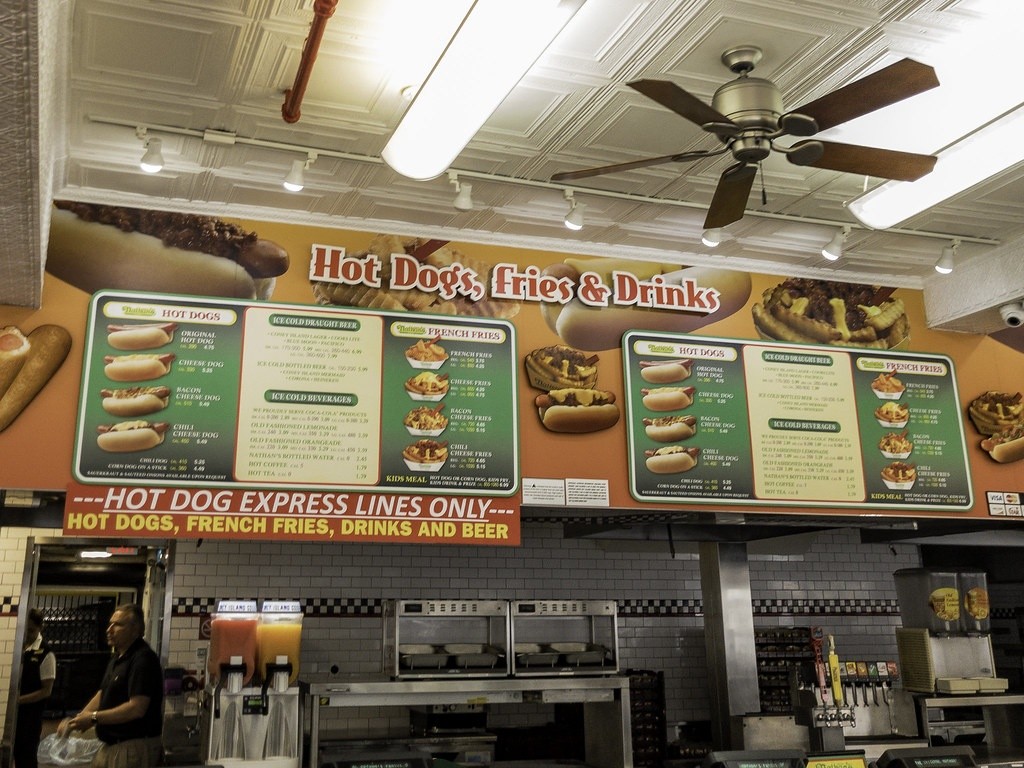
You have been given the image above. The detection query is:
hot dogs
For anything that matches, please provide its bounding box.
[539,256,753,351]
[981,425,1024,464]
[638,358,700,473]
[534,388,620,433]
[45,201,290,302]
[96,322,178,453]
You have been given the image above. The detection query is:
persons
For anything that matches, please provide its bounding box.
[11,610,56,768]
[59,604,167,768]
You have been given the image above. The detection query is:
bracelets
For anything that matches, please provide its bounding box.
[91,709,99,725]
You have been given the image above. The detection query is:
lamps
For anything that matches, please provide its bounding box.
[448,173,475,214]
[135,127,166,175]
[282,152,319,194]
[559,193,588,233]
[820,226,854,262]
[934,240,965,276]
[700,224,725,250]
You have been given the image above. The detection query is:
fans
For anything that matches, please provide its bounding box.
[547,41,944,234]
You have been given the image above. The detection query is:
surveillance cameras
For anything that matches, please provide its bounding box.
[1000,303,1024,328]
